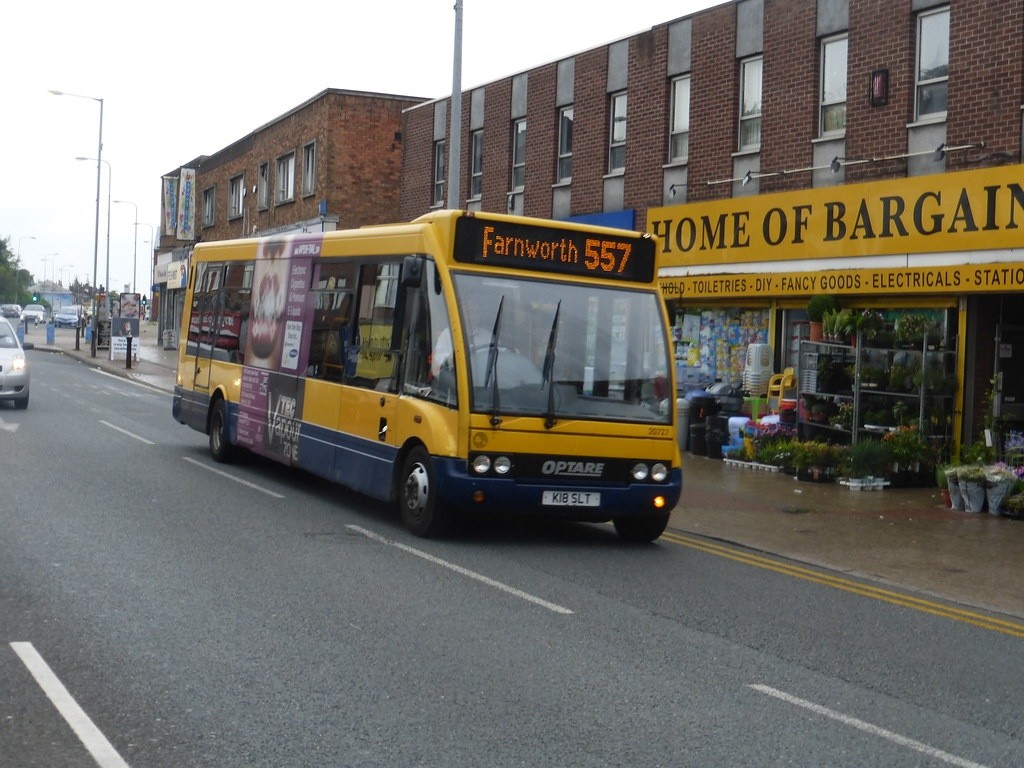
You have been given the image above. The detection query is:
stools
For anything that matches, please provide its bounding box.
[743,359,769,392]
[743,353,769,386]
[743,348,773,378]
[744,363,768,395]
[743,344,774,375]
[743,350,772,383]
[743,356,769,389]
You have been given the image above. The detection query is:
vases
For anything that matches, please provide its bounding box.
[898,340,940,351]
[886,458,913,483]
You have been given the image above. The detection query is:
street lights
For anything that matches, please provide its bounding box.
[49,89,104,351]
[13,236,36,303]
[134,222,154,321]
[76,156,112,321]
[113,199,138,343]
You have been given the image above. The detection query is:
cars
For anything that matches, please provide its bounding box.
[0,314,34,410]
[0,303,98,329]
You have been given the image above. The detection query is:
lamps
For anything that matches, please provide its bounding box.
[742,170,784,187]
[932,143,981,161]
[669,182,707,199]
[831,156,873,173]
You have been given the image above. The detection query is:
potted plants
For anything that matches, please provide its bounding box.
[723,293,1024,519]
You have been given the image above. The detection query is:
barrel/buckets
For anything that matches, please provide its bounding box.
[779,398,797,423]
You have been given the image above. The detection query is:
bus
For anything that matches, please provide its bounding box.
[172,206,684,546]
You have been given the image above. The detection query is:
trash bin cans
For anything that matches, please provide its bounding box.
[685,390,714,450]
[704,416,729,459]
[660,398,690,452]
[705,382,731,398]
[689,423,707,455]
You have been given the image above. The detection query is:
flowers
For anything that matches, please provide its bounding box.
[743,436,762,460]
[944,466,966,510]
[900,312,943,343]
[983,465,1018,516]
[830,401,853,424]
[931,416,951,467]
[956,466,988,513]
[880,424,943,466]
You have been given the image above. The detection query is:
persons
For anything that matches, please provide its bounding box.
[181,265,186,287]
[430,303,520,377]
[119,321,132,337]
[82,313,88,327]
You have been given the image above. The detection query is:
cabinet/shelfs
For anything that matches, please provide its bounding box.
[854,332,958,444]
[796,317,898,445]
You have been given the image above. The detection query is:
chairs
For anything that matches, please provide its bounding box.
[769,367,798,404]
[767,366,799,406]
